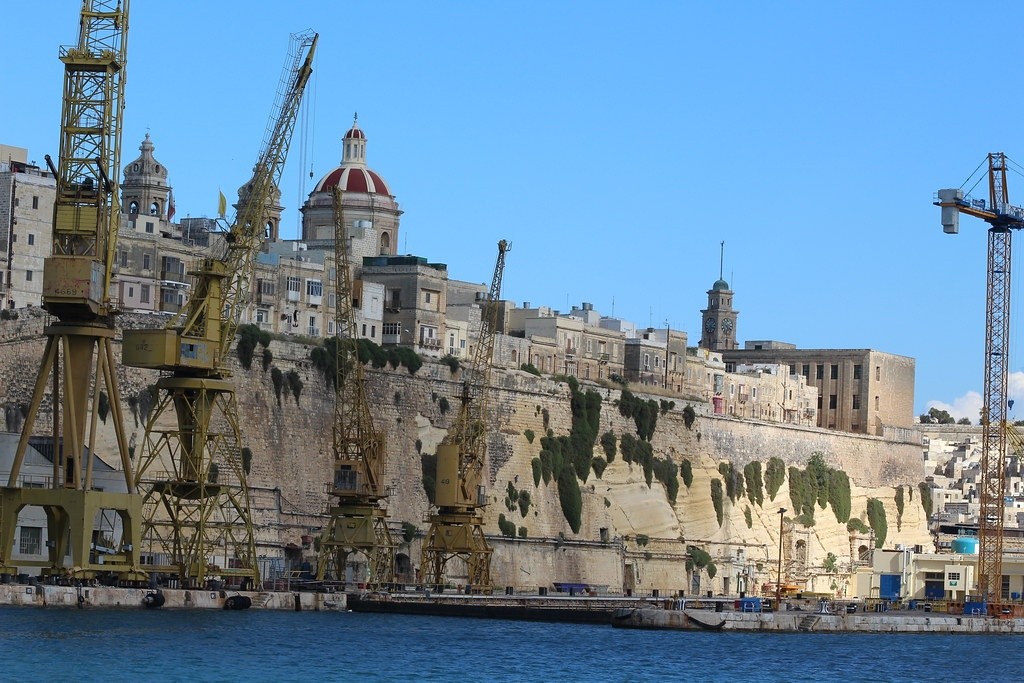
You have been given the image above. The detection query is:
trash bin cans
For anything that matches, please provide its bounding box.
[739,598,760,613]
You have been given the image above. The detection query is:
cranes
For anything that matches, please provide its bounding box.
[931,151,1024,617]
